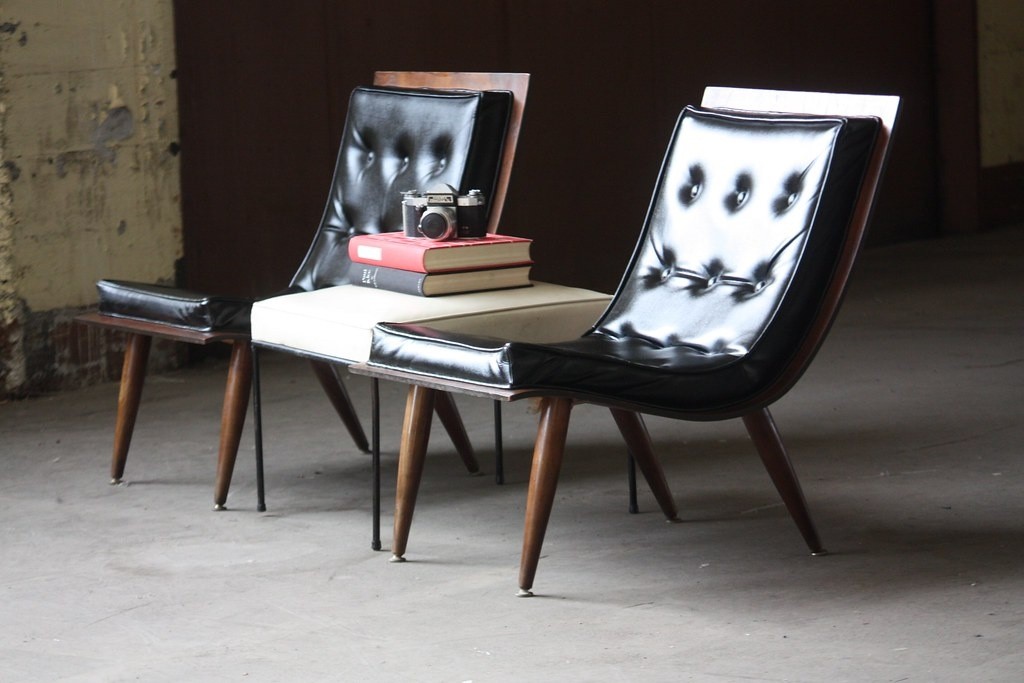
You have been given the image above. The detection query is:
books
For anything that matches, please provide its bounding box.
[348,262,533,296]
[348,231,535,273]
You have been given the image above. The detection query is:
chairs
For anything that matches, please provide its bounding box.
[73,71,535,514]
[349,86,902,598]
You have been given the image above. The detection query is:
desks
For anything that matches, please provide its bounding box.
[249,279,638,551]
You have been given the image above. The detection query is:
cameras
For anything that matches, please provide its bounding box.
[401,182,487,242]
